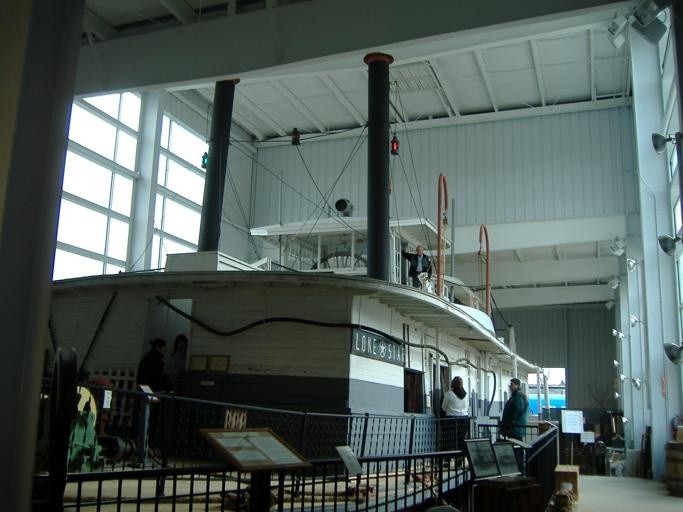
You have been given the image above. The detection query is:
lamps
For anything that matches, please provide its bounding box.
[390,81,399,156]
[656,231,681,255]
[605,239,645,426]
[200,152,208,168]
[651,133,678,152]
[661,340,682,364]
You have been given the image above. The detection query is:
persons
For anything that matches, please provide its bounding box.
[441,375,470,467]
[401,244,432,287]
[136,334,189,392]
[500,378,527,476]
